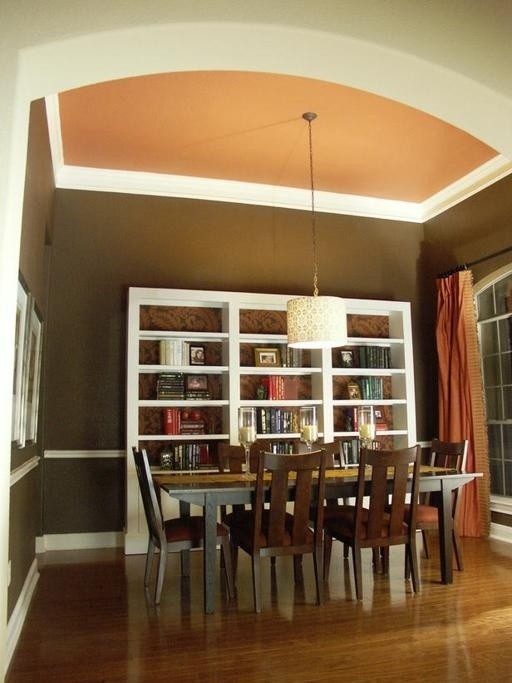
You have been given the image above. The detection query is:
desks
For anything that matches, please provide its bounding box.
[148,462,486,615]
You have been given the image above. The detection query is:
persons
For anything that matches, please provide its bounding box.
[261,355,273,362]
[343,353,352,367]
[186,376,201,388]
[194,349,203,361]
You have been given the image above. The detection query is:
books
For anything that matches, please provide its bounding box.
[171,443,219,470]
[258,374,298,454]
[184,391,214,401]
[358,345,392,369]
[157,338,190,366]
[160,407,205,435]
[343,405,386,466]
[358,375,384,400]
[278,343,304,367]
[154,373,185,400]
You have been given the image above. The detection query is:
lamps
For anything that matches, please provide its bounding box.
[286,111,348,351]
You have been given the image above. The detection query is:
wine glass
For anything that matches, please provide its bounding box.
[298,405,317,454]
[236,405,258,478]
[358,404,376,450]
